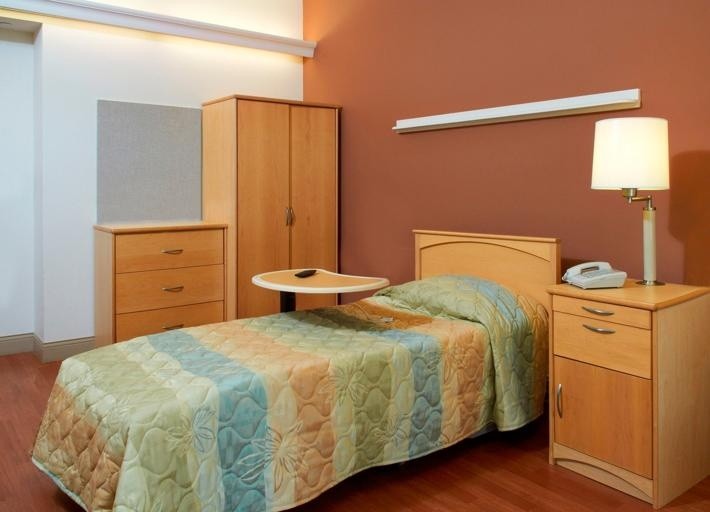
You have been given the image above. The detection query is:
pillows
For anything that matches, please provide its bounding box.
[377,276,544,322]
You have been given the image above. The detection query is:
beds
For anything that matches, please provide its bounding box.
[31,228,561,511]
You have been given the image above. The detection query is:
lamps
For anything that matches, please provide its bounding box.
[591,116,671,286]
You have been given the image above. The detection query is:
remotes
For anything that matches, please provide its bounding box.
[294,270,318,277]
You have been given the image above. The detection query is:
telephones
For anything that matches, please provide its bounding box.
[561,261,627,289]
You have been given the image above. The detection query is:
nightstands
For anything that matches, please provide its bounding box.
[549,276,710,509]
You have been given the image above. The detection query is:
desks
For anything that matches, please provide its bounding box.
[252,269,390,313]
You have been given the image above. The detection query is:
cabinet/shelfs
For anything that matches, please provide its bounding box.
[92,222,228,348]
[201,95,341,321]
